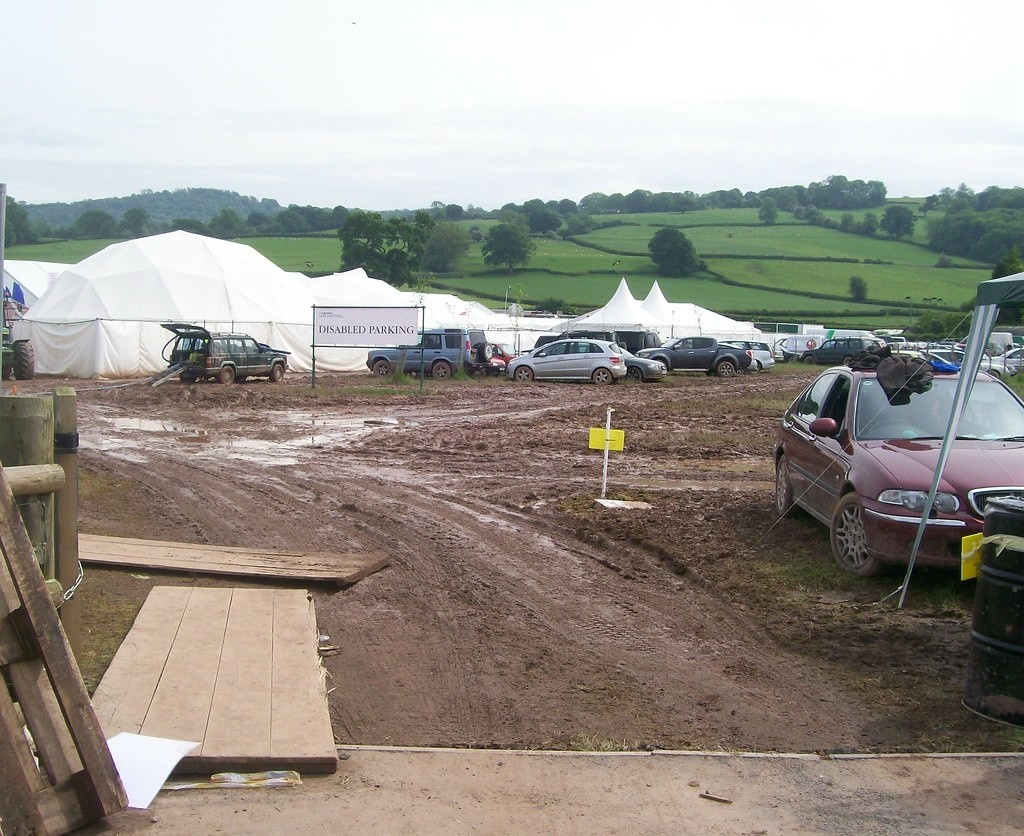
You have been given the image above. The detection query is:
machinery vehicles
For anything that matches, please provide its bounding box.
[2,291,35,380]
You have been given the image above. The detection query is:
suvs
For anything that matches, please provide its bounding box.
[800,338,885,365]
[366,329,493,379]
[160,324,290,386]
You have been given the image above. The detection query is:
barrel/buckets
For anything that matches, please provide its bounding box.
[960,495,1024,729]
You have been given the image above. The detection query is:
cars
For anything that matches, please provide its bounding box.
[772,365,1024,578]
[488,343,517,373]
[879,335,1024,380]
[771,344,784,361]
[506,339,627,385]
[718,341,775,374]
[619,347,668,382]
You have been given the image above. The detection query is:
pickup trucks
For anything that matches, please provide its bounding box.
[634,337,752,378]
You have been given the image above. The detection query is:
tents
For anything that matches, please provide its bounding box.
[2,229,762,378]
